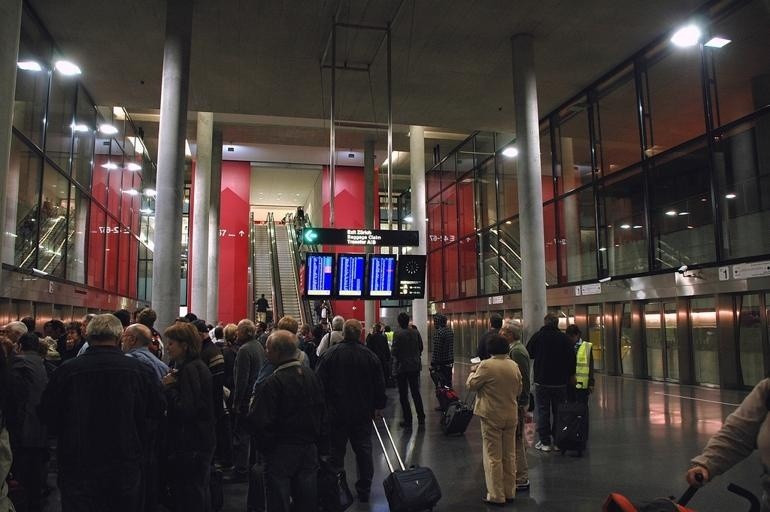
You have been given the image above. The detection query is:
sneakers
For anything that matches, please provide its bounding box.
[535,441,551,452]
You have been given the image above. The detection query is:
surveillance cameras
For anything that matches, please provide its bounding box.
[678,265,688,274]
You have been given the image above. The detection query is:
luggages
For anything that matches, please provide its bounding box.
[440,392,478,437]
[554,382,586,457]
[371,414,442,512]
[429,367,458,411]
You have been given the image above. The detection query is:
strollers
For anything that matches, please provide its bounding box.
[603,473,760,512]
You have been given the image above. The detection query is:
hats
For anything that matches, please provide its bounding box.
[190,320,209,332]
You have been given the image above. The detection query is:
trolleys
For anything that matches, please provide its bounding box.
[429,368,462,409]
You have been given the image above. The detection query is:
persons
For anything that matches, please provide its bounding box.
[429,311,455,412]
[476,313,502,361]
[296,205,316,262]
[526,314,575,451]
[314,299,326,317]
[18,196,56,243]
[497,319,530,491]
[465,335,523,507]
[253,293,270,322]
[564,324,594,436]
[317,302,331,316]
[0,310,424,512]
[687,377,769,511]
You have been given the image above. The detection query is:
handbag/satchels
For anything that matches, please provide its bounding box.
[317,466,353,511]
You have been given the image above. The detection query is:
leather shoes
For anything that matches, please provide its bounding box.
[222,471,250,483]
